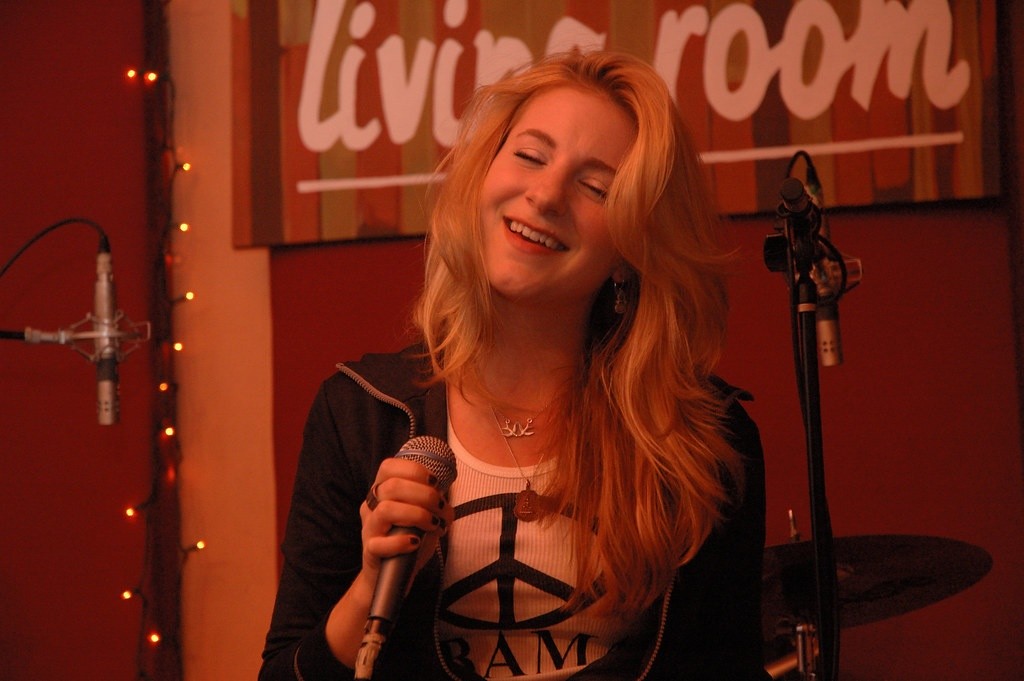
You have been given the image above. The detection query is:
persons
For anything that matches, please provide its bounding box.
[258,53,765,680]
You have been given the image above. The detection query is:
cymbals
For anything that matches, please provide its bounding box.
[761,533,993,635]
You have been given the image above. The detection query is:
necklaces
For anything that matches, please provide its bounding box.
[485,398,556,523]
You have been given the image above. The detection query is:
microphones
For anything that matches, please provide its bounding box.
[354,437,458,681]
[93,235,119,425]
[804,167,843,366]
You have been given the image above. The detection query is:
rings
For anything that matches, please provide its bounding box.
[366,483,378,510]
